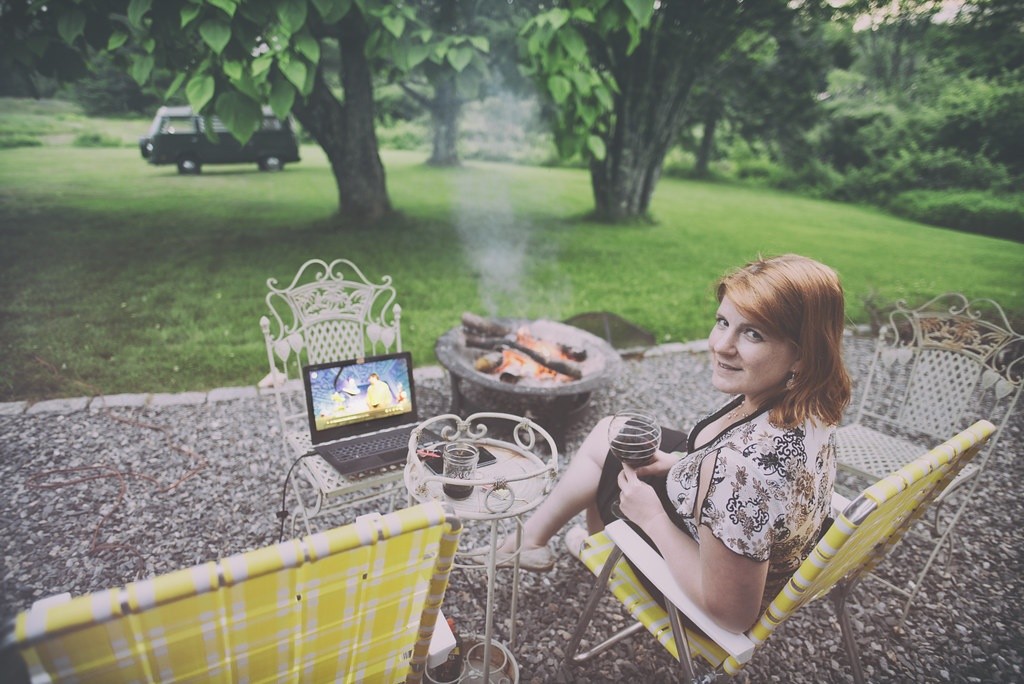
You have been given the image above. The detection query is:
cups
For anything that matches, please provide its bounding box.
[442,443,479,501]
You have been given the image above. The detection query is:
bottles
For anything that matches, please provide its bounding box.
[435,617,463,684]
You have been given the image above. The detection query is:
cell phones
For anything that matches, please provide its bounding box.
[425,446,496,476]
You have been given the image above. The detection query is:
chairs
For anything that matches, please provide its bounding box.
[564,420,997,684]
[829,293,1024,640]
[0,497,457,684]
[260,258,415,538]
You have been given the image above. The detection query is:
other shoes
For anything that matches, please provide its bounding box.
[564,525,590,558]
[468,536,556,573]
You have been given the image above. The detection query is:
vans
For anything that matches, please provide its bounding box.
[140,103,302,175]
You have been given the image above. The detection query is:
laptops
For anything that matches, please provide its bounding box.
[303,351,448,477]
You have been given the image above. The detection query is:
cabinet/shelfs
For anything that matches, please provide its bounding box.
[402,411,558,684]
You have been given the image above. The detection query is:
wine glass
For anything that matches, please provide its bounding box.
[608,409,662,522]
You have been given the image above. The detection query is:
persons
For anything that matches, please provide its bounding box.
[365,374,392,409]
[466,250,852,636]
